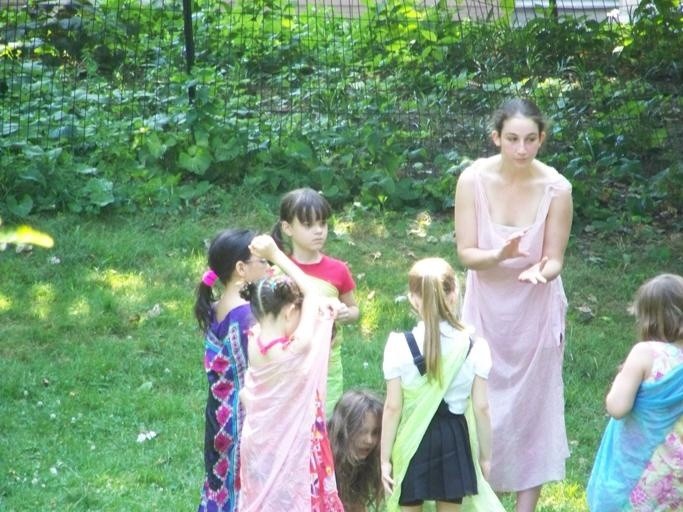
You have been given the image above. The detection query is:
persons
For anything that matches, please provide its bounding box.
[266,185,360,419]
[379,258,505,512]
[194,229,270,512]
[323,386,386,512]
[583,274,683,512]
[235,235,346,512]
[454,96,574,512]
